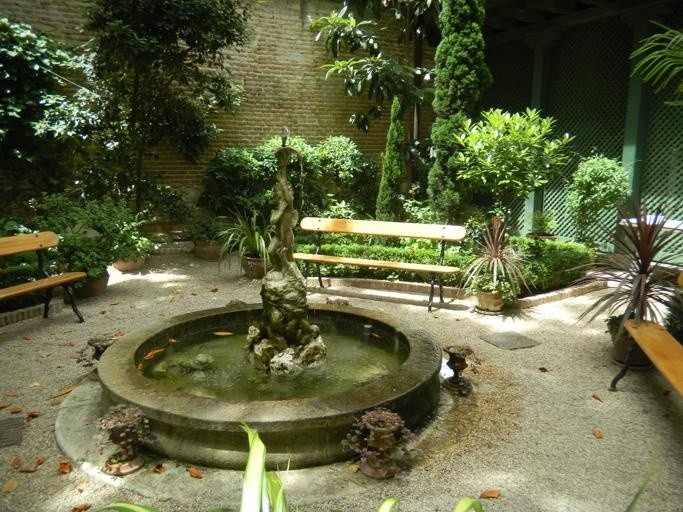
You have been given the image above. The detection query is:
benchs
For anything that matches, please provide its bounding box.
[292,215,467,312]
[1,230,87,323]
[609,273,682,396]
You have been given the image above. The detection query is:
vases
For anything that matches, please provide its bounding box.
[111,433,139,459]
[350,441,398,477]
[444,347,474,391]
[87,338,113,360]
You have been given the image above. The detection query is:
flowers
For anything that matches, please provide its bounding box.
[344,406,421,476]
[93,404,155,460]
[442,342,482,396]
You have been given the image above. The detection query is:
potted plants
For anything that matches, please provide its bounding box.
[455,216,538,315]
[189,205,277,279]
[68,217,151,298]
[565,196,683,371]
